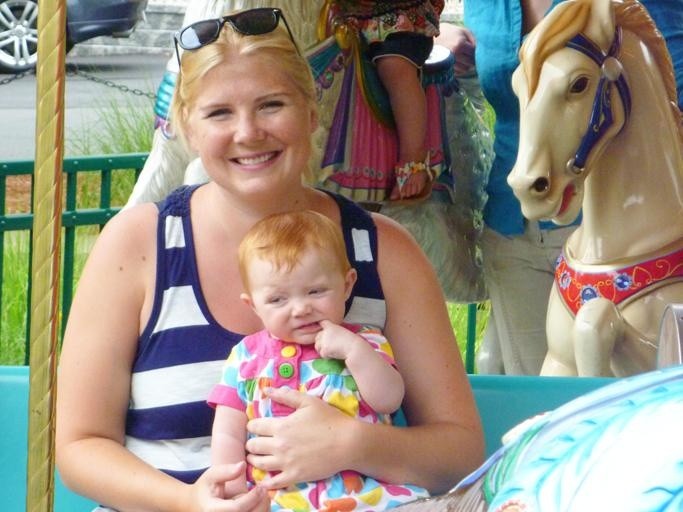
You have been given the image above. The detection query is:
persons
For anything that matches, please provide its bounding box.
[54,5,485,512]
[327,1,445,207]
[435,0,683,377]
[205,211,435,511]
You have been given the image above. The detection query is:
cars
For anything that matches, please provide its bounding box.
[0,0,147,75]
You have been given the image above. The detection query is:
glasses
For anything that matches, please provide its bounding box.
[173,8,300,67]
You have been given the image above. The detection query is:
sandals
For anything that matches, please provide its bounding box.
[389,150,436,205]
